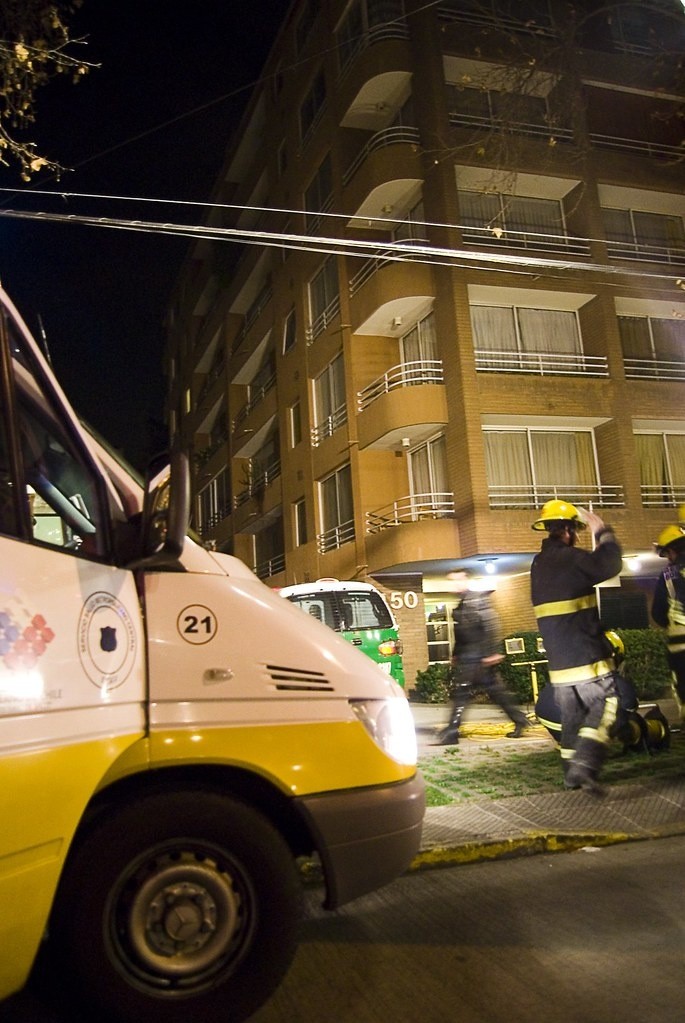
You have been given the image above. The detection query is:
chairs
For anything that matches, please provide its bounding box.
[308,605,323,621]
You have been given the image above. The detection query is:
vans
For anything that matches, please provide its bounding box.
[271,578,406,692]
[0,284,427,1023]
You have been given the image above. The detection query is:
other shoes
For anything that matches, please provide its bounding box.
[506,716,532,738]
[428,734,458,747]
[564,771,605,800]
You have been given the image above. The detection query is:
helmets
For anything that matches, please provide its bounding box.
[531,499,586,531]
[657,524,685,557]
[678,505,685,523]
[604,631,624,655]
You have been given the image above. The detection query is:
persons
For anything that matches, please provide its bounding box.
[428,567,531,746]
[529,500,624,798]
[535,631,637,751]
[651,504,685,731]
